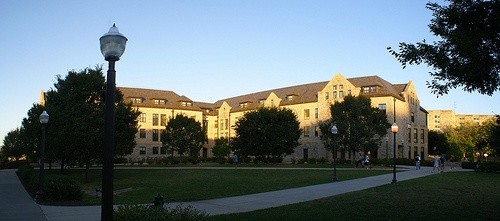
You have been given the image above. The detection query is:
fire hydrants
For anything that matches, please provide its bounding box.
[152,192,164,207]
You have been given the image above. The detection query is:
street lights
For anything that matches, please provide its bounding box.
[39,110,49,177]
[331,125,338,179]
[99,23,129,221]
[390,123,399,184]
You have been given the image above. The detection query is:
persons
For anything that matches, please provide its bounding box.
[415,154,421,170]
[431,153,445,173]
[232,153,240,168]
[355,153,370,170]
